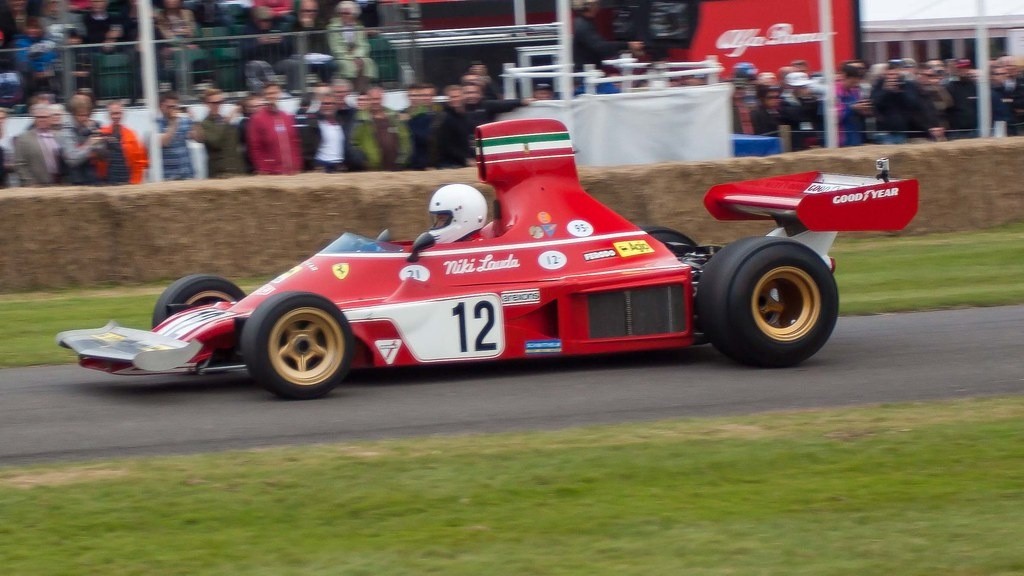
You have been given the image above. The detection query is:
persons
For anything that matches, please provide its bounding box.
[158,92,199,180]
[0,0,1024,186]
[90,102,148,184]
[751,85,802,150]
[246,82,301,173]
[199,86,244,179]
[14,102,66,187]
[428,183,488,243]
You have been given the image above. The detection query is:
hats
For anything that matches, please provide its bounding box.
[889,59,914,70]
[786,71,815,85]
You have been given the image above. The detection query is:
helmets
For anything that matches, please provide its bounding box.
[428,183,487,243]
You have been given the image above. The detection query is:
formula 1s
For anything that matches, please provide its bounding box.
[55,117,921,399]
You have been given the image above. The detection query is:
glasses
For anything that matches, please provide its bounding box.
[764,94,780,99]
[209,99,226,104]
